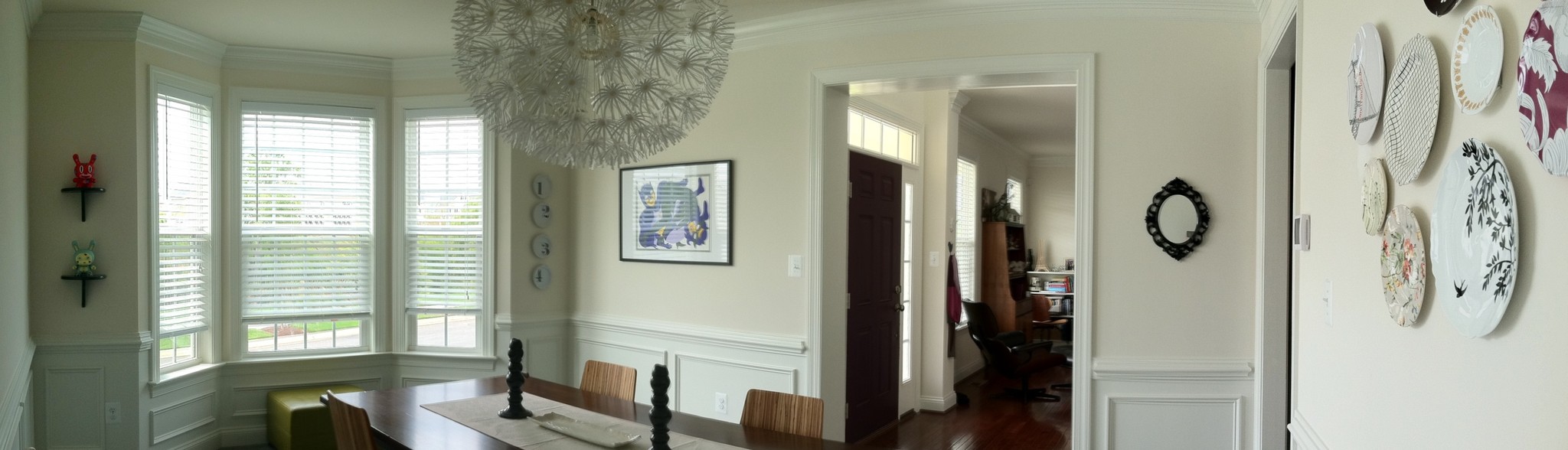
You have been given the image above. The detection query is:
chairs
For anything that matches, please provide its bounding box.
[580,359,637,402]
[961,297,1062,420]
[1032,296,1069,345]
[738,389,825,440]
[326,389,377,449]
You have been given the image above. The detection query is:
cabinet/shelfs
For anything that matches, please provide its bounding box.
[982,220,1034,333]
[1028,271,1073,355]
[60,153,106,310]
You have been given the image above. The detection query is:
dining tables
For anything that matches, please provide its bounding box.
[319,373,851,449]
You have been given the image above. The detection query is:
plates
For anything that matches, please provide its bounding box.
[1344,0,1568,338]
[527,411,641,448]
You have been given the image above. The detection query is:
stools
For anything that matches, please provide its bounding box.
[267,385,365,450]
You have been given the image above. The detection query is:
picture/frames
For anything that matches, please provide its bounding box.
[619,158,734,268]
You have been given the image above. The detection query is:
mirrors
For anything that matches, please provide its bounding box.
[1144,176,1210,261]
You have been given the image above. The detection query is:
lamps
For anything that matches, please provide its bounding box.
[448,1,735,170]
